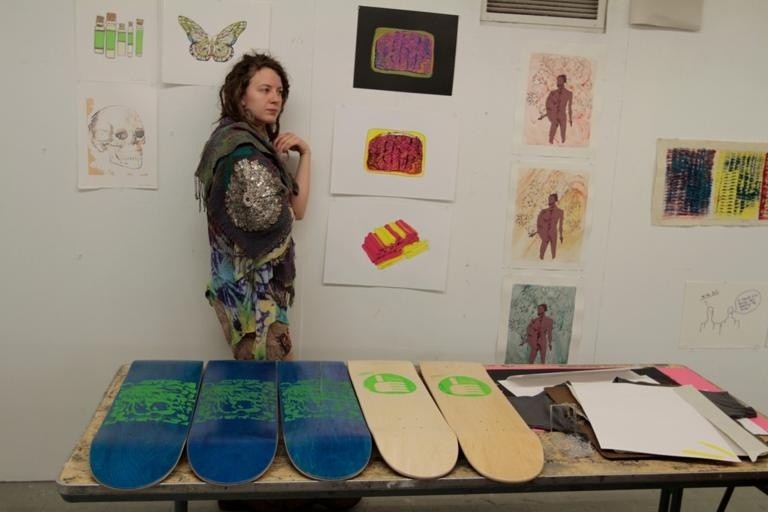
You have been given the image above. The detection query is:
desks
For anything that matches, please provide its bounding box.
[53,361,768,512]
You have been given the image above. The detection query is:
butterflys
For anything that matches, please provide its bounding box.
[176,15,248,64]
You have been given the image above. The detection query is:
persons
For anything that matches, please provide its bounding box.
[537,193,564,260]
[194,53,311,361]
[546,74,572,144]
[527,304,552,364]
